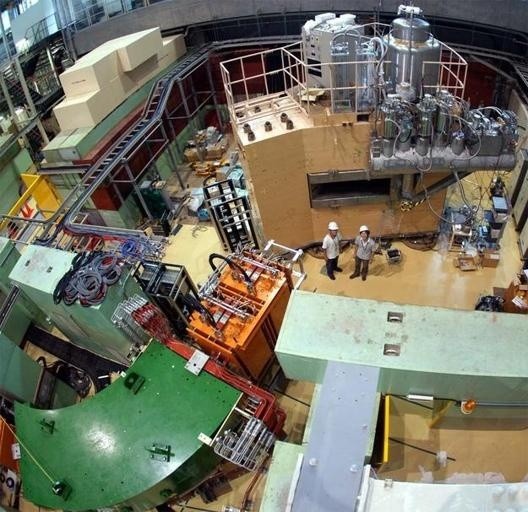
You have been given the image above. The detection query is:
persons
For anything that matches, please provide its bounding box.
[349,226,376,281]
[321,222,343,281]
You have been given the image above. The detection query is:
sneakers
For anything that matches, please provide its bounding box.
[350,274,360,279]
[328,274,335,280]
[334,267,342,272]
[362,275,366,281]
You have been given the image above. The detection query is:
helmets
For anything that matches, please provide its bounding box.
[359,225,370,232]
[328,222,339,231]
